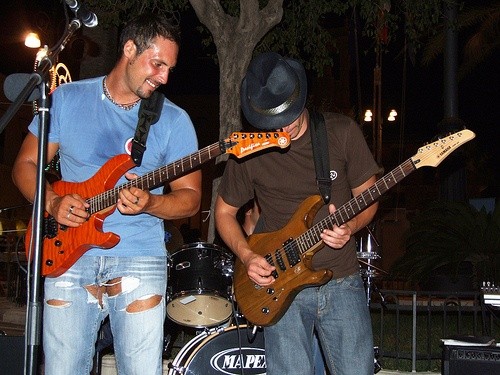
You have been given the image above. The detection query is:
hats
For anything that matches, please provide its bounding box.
[239,52,308,130]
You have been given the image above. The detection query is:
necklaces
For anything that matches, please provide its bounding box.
[103,78,140,110]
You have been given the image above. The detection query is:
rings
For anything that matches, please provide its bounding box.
[135,197,139,204]
[67,206,74,218]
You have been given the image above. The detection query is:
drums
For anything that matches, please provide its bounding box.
[166,241,234,329]
[167,324,267,375]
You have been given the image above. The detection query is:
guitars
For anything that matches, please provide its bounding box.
[25,128,292,279]
[233,128,476,327]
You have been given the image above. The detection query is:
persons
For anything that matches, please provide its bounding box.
[240,197,261,238]
[214,52,379,375]
[11,16,202,375]
